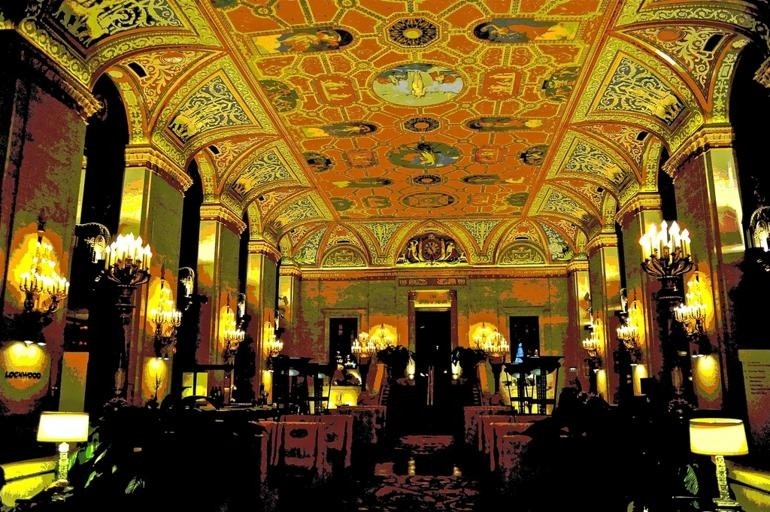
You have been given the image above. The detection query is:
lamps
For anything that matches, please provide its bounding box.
[152,308,183,358]
[103,230,153,325]
[688,416,750,508]
[615,324,641,354]
[221,328,247,363]
[581,331,597,358]
[35,410,91,494]
[673,299,708,345]
[637,219,695,300]
[18,268,40,315]
[265,334,285,370]
[42,273,71,313]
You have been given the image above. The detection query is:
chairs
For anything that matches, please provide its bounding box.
[71,395,552,511]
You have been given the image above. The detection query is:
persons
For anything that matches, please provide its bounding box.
[481,23,573,42]
[273,30,340,52]
[406,240,418,261]
[445,241,456,260]
[411,71,427,100]
